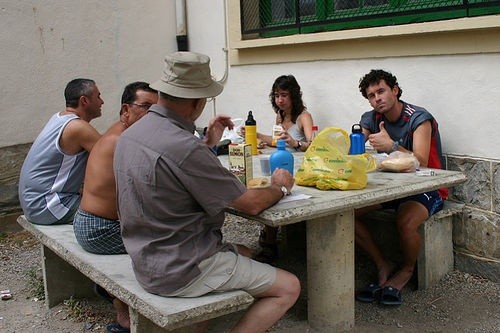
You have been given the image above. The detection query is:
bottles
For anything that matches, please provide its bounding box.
[309,125,318,147]
[245,110,258,155]
[349,123,366,154]
[269,139,295,175]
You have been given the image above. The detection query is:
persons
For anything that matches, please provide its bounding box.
[72,81,159,333]
[240,75,314,247]
[113,51,300,333]
[354,69,448,305]
[18,79,104,225]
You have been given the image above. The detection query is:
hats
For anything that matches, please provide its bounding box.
[149,51,224,98]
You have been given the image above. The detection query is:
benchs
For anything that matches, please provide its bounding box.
[17,215,255,333]
[368,199,466,288]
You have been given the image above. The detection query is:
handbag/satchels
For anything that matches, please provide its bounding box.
[220,119,245,144]
[294,127,376,190]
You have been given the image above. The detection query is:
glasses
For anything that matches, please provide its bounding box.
[127,103,151,110]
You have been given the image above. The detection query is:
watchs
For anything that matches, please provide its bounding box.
[274,182,287,198]
[297,141,301,149]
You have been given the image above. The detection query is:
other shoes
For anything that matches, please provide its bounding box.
[255,246,278,262]
[259,225,278,246]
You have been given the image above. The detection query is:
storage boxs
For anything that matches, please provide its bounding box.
[228,143,253,188]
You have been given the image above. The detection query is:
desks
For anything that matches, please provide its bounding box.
[215,143,466,333]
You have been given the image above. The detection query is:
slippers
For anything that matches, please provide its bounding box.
[94,284,113,305]
[356,282,381,301]
[107,325,130,333]
[377,287,401,305]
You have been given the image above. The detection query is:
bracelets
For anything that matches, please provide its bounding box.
[392,141,399,150]
